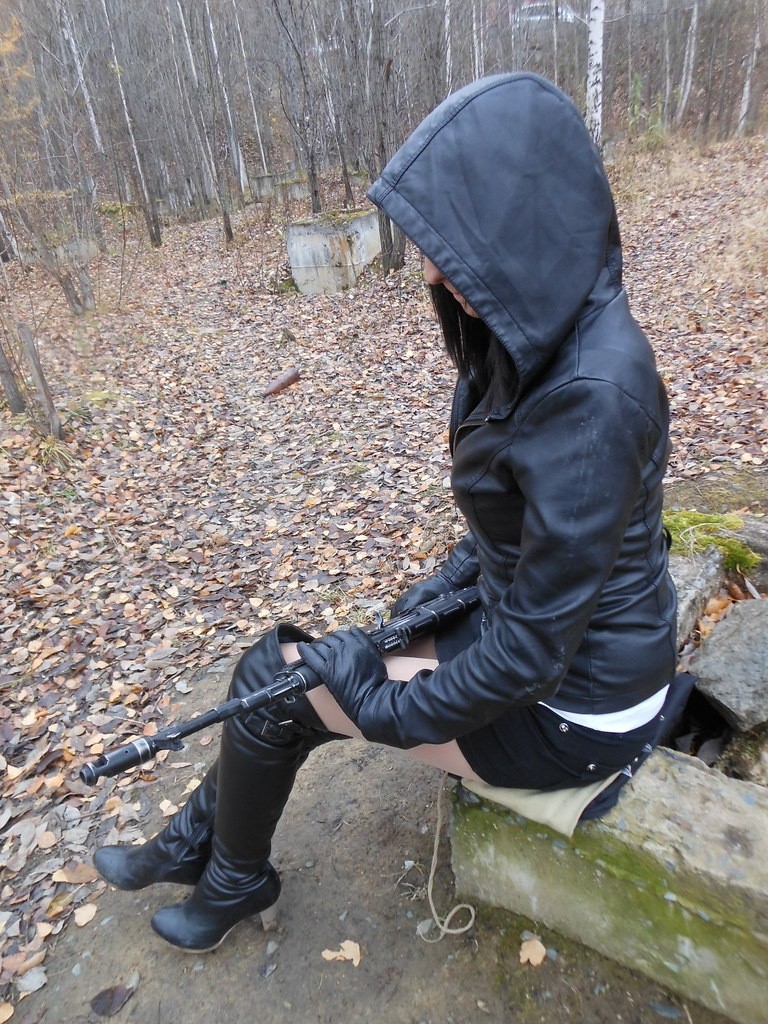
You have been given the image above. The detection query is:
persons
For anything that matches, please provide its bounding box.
[87,73,679,950]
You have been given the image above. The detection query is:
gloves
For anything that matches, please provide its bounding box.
[389,571,458,621]
[296,624,389,732]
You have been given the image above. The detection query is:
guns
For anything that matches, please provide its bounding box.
[79,526,672,788]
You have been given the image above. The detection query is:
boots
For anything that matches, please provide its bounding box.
[91,733,353,893]
[151,621,354,954]
